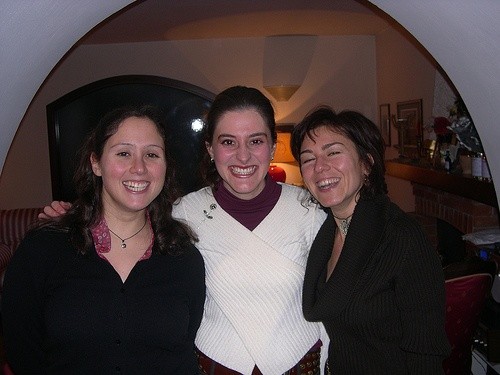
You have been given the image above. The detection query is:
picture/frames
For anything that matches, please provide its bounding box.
[379,104,391,147]
[396,98,423,154]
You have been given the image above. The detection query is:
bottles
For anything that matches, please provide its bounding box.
[432,140,441,168]
[444,151,451,171]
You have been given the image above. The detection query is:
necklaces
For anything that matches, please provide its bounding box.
[108,217,147,248]
[333,214,353,236]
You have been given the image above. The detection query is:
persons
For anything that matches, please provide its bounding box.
[0,106,207,375]
[290,105,450,375]
[39,85,331,375]
